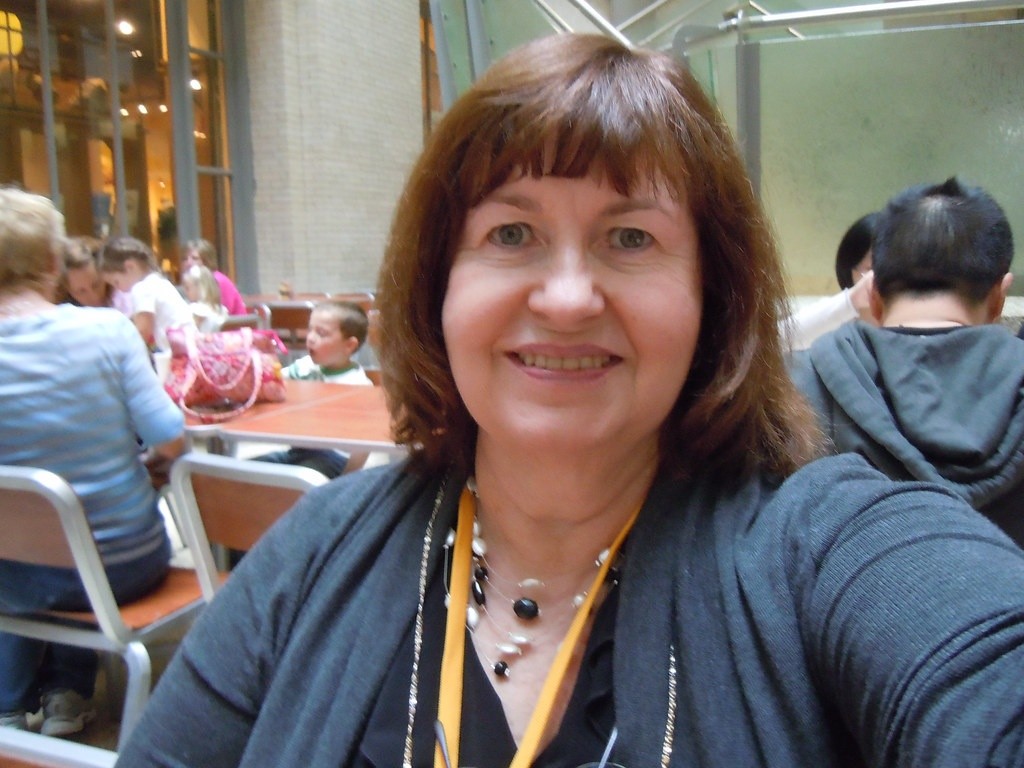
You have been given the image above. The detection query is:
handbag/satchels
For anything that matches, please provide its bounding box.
[160,323,288,421]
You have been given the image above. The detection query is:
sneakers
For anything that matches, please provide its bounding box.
[40,690,96,735]
[0,711,28,732]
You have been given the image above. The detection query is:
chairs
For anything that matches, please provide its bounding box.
[0,292,382,768]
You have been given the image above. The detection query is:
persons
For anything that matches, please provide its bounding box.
[228,299,375,570]
[49,235,133,319]
[182,264,228,336]
[178,237,249,332]
[774,211,884,354]
[113,31,1023,768]
[781,177,1024,550]
[0,185,188,738]
[98,236,202,489]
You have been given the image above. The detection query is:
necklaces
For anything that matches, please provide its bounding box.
[441,477,611,679]
[401,495,678,768]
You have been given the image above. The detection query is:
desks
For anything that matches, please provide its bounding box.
[167,381,420,569]
